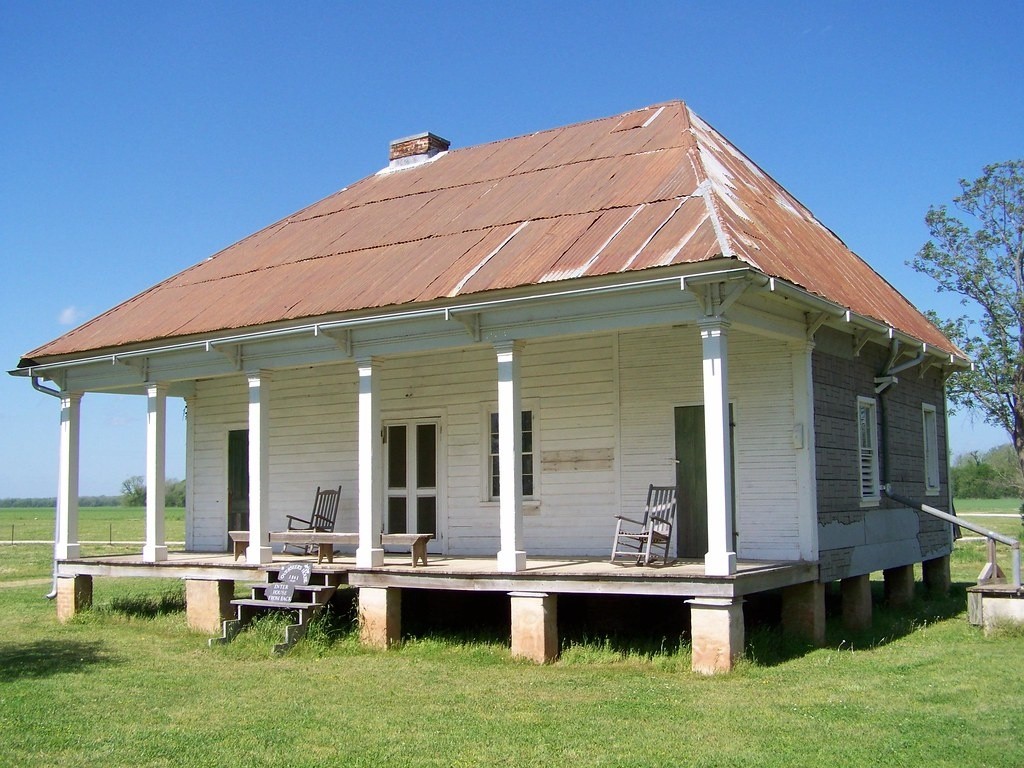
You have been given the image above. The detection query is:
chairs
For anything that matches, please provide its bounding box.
[611,483,680,568]
[282,485,342,558]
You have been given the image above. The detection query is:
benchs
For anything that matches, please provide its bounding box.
[228,530,434,567]
